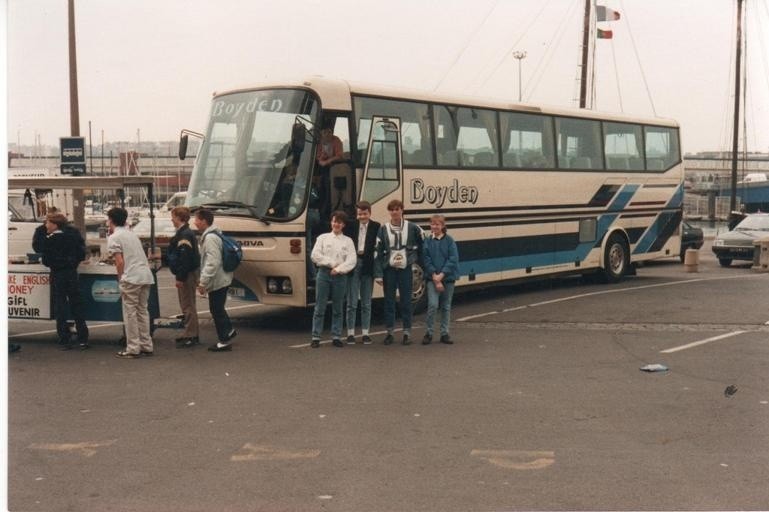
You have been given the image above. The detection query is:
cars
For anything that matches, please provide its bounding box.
[710,212,769,266]
[636,221,706,267]
[10,191,224,269]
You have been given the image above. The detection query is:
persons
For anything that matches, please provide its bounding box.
[373,201,422,345]
[311,211,358,348]
[9,342,21,353]
[32,206,84,258]
[344,201,380,346]
[107,208,154,358]
[422,216,460,345]
[41,214,93,349]
[166,207,201,349]
[315,124,344,198]
[194,211,237,352]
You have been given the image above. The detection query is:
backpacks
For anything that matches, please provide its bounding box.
[201,232,243,273]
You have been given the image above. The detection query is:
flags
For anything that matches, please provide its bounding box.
[597,28,612,40]
[596,4,620,21]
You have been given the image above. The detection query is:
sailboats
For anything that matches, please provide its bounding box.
[12,121,184,233]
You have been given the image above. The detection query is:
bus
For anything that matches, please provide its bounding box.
[175,83,687,316]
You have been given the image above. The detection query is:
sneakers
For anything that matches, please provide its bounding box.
[175,335,197,349]
[114,350,153,358]
[310,334,454,347]
[80,341,90,350]
[61,344,71,351]
[208,342,232,352]
[227,328,240,342]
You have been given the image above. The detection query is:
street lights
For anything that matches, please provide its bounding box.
[511,46,528,104]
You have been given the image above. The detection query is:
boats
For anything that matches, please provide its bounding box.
[93,288,120,298]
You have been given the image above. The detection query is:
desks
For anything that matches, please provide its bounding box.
[8,261,159,345]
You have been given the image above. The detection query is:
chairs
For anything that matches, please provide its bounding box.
[343,148,665,172]
